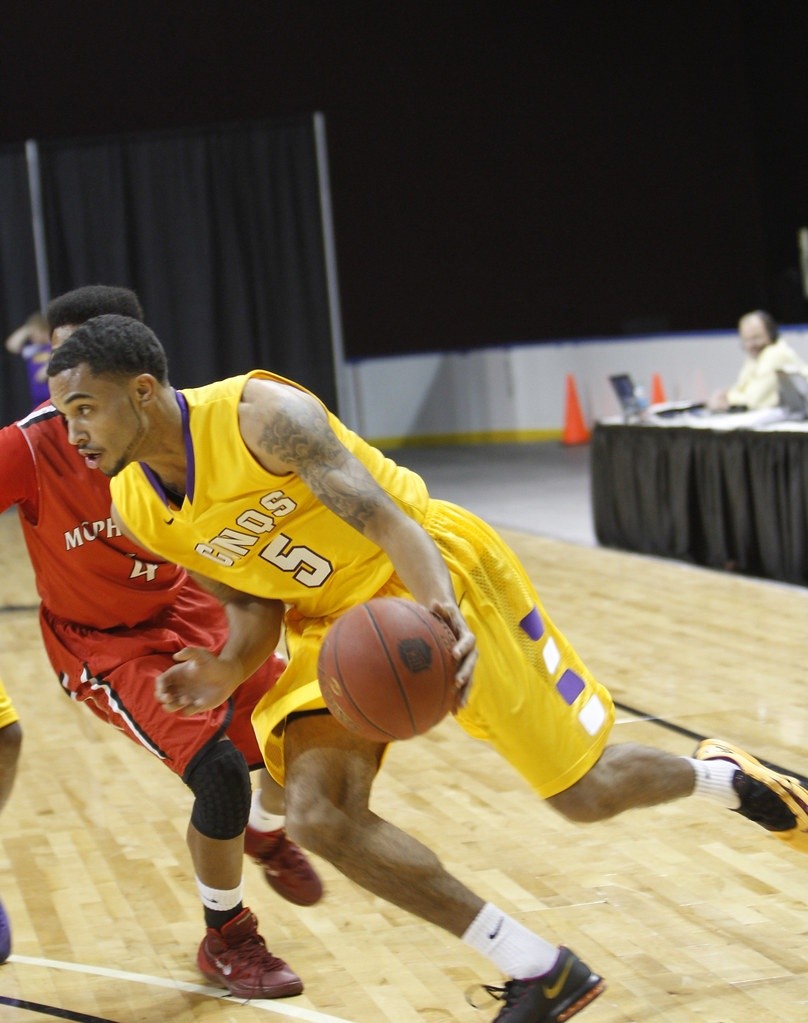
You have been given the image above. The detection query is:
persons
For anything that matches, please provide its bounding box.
[0,285,323,999]
[46,313,808,1023]
[5,313,51,408]
[708,311,808,413]
[0,682,23,967]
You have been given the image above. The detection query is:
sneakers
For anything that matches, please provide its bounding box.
[466,945,607,1023]
[693,738,808,853]
[243,825,322,907]
[197,909,304,999]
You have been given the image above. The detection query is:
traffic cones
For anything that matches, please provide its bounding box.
[555,375,591,447]
[648,374,665,407]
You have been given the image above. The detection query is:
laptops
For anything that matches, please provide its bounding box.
[777,369,808,420]
[611,374,705,418]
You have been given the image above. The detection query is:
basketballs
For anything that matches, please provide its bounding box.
[316,598,463,746]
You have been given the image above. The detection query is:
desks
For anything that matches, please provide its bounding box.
[590,412,808,588]
